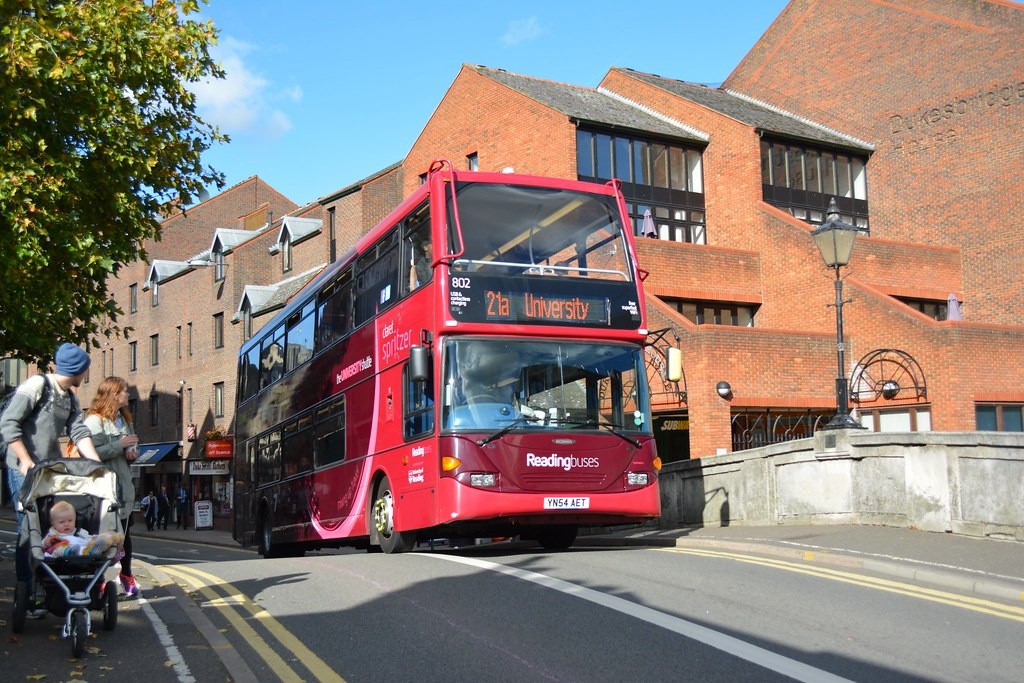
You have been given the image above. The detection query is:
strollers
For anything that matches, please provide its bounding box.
[15,458,125,658]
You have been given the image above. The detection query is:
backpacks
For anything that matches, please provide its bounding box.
[0,373,75,462]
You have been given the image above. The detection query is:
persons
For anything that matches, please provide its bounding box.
[0,343,102,618]
[140,481,191,531]
[41,501,125,559]
[67,377,139,596]
[416,234,433,286]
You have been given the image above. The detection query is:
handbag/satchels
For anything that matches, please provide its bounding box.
[140,496,149,513]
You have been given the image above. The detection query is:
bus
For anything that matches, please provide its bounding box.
[229,159,684,559]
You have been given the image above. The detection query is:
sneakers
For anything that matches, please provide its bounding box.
[118,570,139,596]
[100,577,107,593]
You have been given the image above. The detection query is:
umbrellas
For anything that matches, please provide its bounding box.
[640,209,657,238]
[947,294,961,320]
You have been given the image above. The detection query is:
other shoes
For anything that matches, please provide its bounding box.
[24,608,49,620]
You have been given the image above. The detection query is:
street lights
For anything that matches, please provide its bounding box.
[809,197,870,431]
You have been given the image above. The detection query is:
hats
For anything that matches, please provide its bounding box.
[55,342,92,377]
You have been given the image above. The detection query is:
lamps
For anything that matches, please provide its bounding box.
[268,244,296,256]
[141,283,151,292]
[187,259,229,266]
[882,382,896,397]
[716,381,731,397]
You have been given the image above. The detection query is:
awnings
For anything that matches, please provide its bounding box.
[129,442,178,467]
